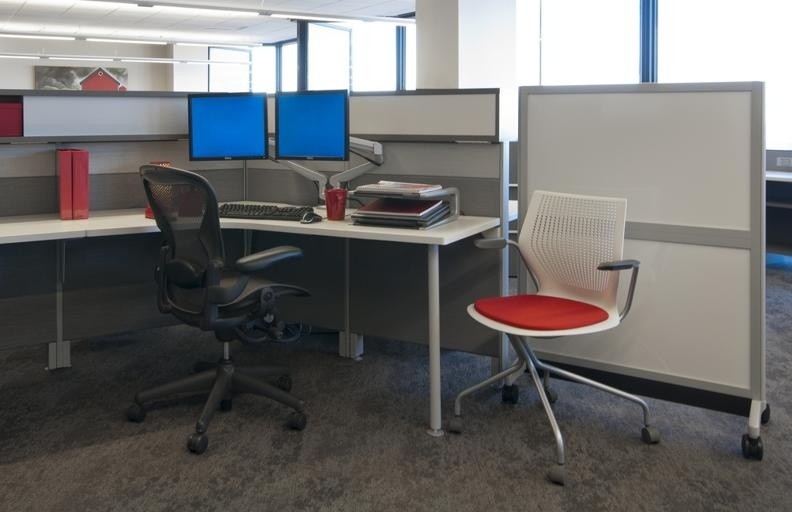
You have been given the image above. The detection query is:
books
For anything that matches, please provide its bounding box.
[350,179,452,227]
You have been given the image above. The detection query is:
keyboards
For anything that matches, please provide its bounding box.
[218,203,314,221]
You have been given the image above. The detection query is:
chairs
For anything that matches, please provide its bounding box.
[449,188,660,485]
[126,163,313,456]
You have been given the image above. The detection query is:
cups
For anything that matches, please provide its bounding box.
[325,188,347,221]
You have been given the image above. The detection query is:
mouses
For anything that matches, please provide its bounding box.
[300,212,322,224]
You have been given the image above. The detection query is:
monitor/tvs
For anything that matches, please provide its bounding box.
[188,93,269,161]
[275,89,350,161]
